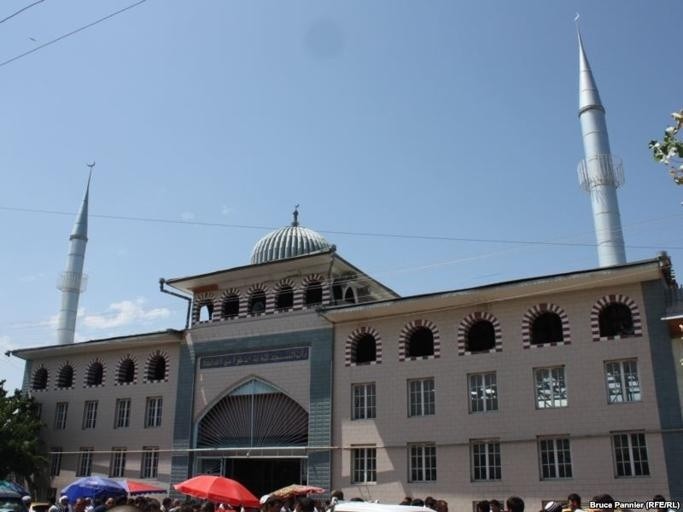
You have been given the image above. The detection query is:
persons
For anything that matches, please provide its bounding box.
[47,489,345,511]
[19,493,31,511]
[474,490,617,511]
[400,496,448,511]
[652,494,676,511]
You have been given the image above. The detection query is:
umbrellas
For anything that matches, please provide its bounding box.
[61,473,326,501]
